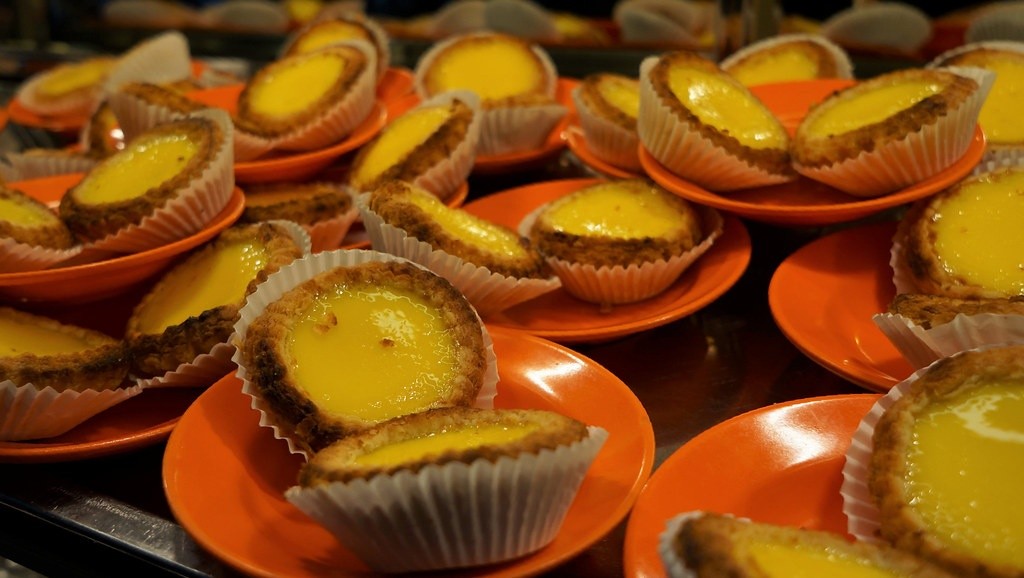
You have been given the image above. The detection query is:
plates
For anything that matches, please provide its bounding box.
[560,114,648,181]
[338,174,468,256]
[184,82,386,187]
[11,59,233,132]
[623,393,902,578]
[375,66,420,108]
[637,79,986,226]
[385,76,582,171]
[0,289,201,461]
[161,324,656,578]
[0,170,245,307]
[456,177,752,343]
[766,218,911,395]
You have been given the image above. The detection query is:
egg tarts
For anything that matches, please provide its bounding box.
[1,0,1024,578]
